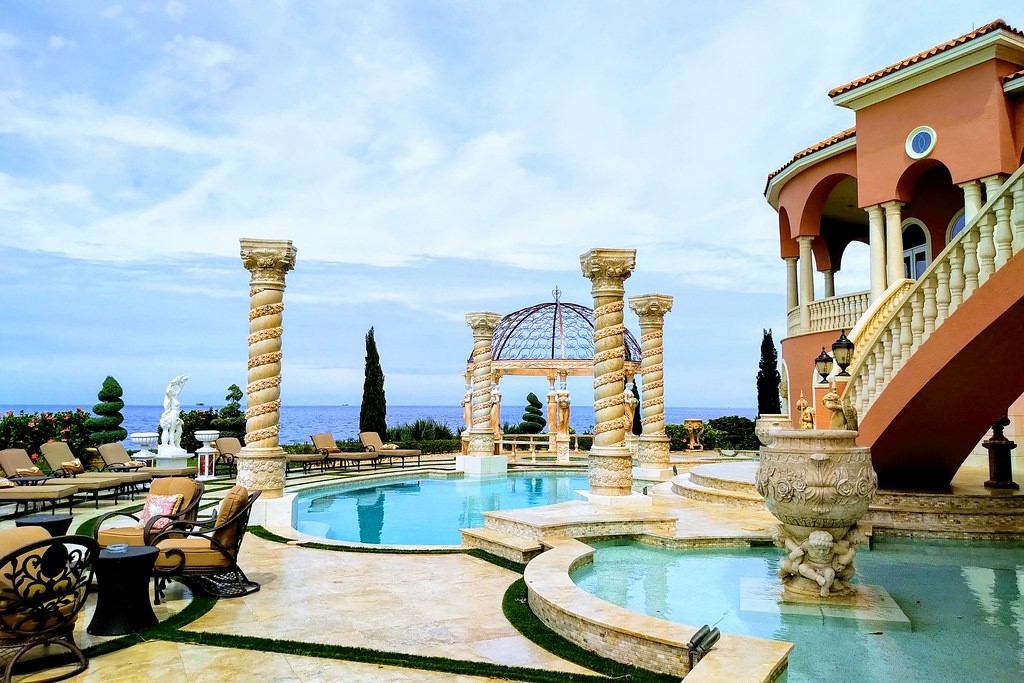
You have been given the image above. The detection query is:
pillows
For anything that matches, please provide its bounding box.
[0,476,16,489]
[324,445,341,453]
[121,460,146,468]
[378,442,397,451]
[60,457,85,474]
[16,466,45,476]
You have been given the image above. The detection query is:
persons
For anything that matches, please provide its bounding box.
[624,383,639,437]
[166,381,184,448]
[796,398,815,429]
[558,391,570,434]
[491,386,501,440]
[461,384,473,435]
[823,393,848,430]
[772,531,856,595]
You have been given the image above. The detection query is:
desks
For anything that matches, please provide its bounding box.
[9,476,53,514]
[86,545,160,637]
[12,513,76,536]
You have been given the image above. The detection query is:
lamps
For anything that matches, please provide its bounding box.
[832,327,856,377]
[814,346,833,383]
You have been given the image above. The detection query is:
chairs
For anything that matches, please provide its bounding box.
[287,450,326,475]
[310,432,380,473]
[95,441,199,490]
[149,485,264,606]
[92,476,209,591]
[357,430,422,469]
[0,448,122,510]
[213,436,242,479]
[40,442,154,501]
[0,525,102,683]
[0,484,80,515]
